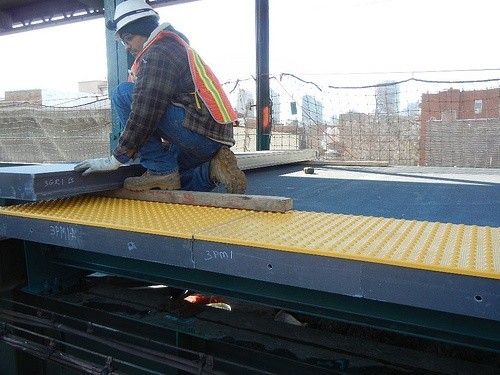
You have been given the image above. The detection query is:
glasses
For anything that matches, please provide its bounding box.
[120,34,138,46]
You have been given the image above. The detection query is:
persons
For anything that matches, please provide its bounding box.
[74,0,247,194]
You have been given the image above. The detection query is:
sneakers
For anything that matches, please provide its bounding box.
[209,147,247,193]
[124,169,182,191]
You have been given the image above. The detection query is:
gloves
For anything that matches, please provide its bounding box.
[74,156,120,176]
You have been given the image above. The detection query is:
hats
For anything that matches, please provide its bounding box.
[113,0,160,39]
